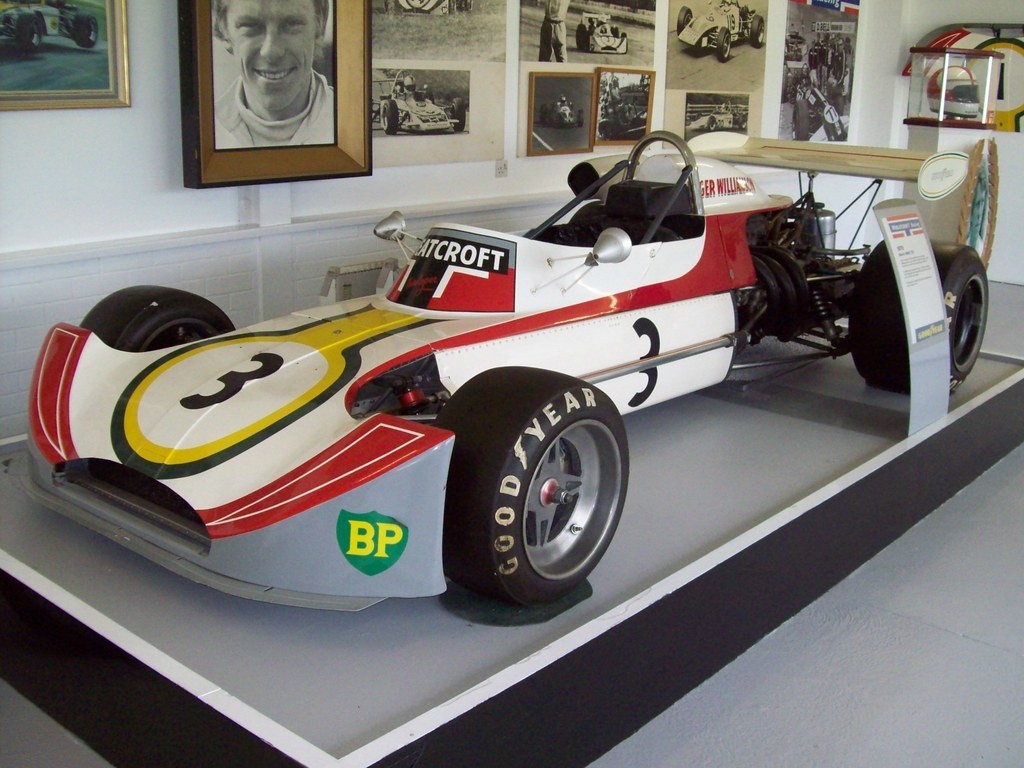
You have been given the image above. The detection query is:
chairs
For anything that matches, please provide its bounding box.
[562,201,684,247]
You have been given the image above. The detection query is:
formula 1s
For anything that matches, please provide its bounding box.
[676,0,765,62]
[575,11,628,54]
[27,136,992,615]
[372,76,466,135]
[0,0,99,54]
[685,105,748,131]
[782,60,847,141]
[539,100,584,128]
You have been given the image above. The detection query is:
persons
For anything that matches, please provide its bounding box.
[399,76,417,102]
[786,30,854,140]
[212,0,335,150]
[539,0,571,62]
[610,79,620,102]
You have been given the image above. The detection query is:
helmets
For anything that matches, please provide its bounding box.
[927,65,980,118]
[404,74,416,91]
[561,97,566,102]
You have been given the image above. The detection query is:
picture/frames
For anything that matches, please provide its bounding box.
[527,71,599,157]
[177,0,373,189]
[0,0,130,111]
[593,67,657,147]
